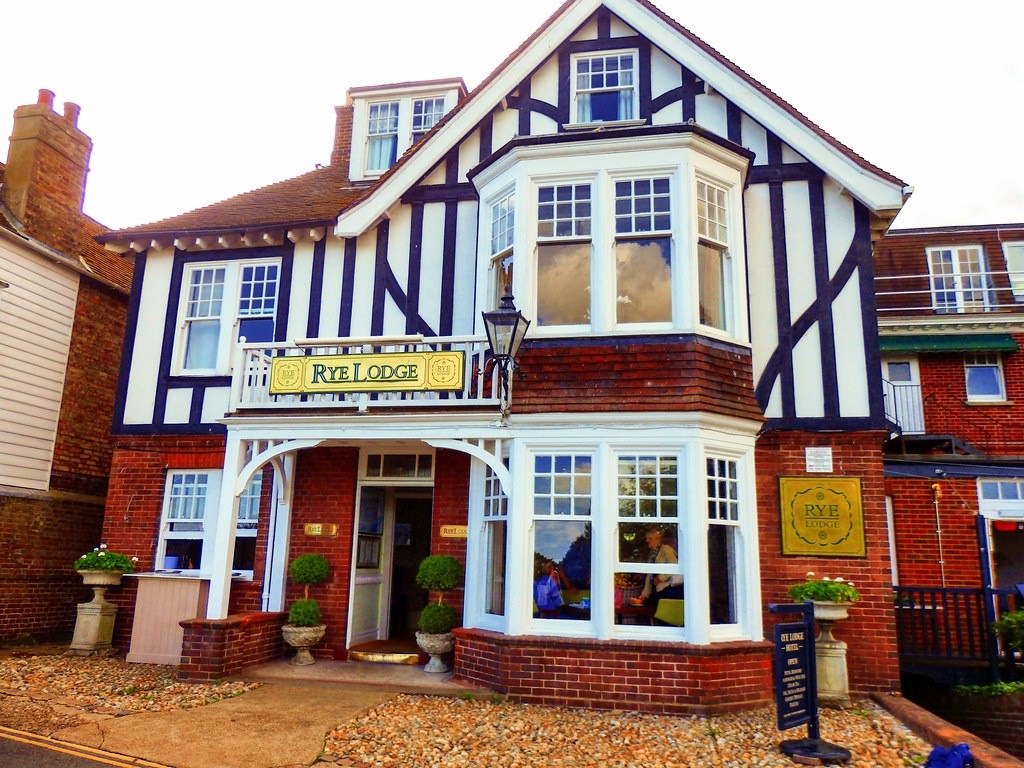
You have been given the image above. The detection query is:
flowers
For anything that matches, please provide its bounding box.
[787,570,859,602]
[73,543,138,574]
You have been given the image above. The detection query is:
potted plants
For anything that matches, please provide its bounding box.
[415,554,464,673]
[282,553,330,666]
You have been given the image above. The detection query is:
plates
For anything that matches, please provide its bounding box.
[631,604,644,607]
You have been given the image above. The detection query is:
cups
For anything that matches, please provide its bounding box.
[632,599,642,604]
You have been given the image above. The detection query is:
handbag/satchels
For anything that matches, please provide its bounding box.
[670,575,683,587]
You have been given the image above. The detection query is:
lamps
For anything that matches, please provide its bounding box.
[475,281,532,402]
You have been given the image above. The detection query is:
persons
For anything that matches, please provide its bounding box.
[530,520,728,623]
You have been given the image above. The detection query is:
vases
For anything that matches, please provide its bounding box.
[813,602,855,708]
[68,570,126,650]
